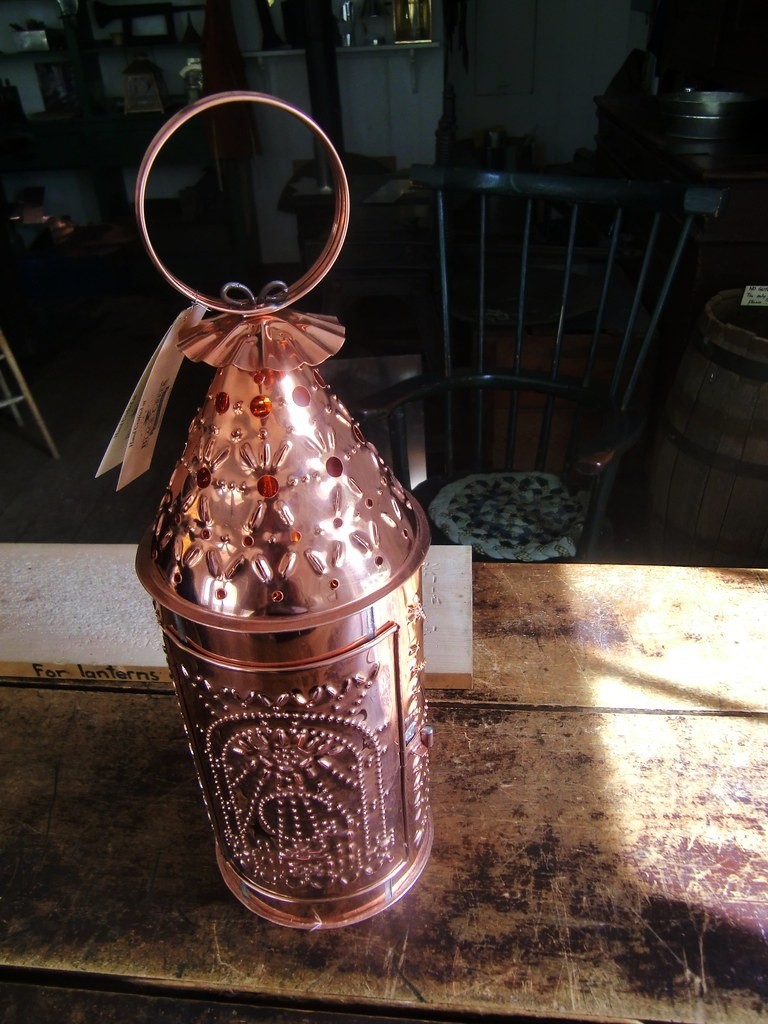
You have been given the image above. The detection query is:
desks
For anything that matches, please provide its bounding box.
[1,560,768,1023]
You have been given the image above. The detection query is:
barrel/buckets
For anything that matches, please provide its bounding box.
[636,289,768,568]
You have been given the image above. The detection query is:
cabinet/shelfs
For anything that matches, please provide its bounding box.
[0,0,260,335]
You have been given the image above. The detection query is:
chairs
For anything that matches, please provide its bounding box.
[381,161,730,563]
